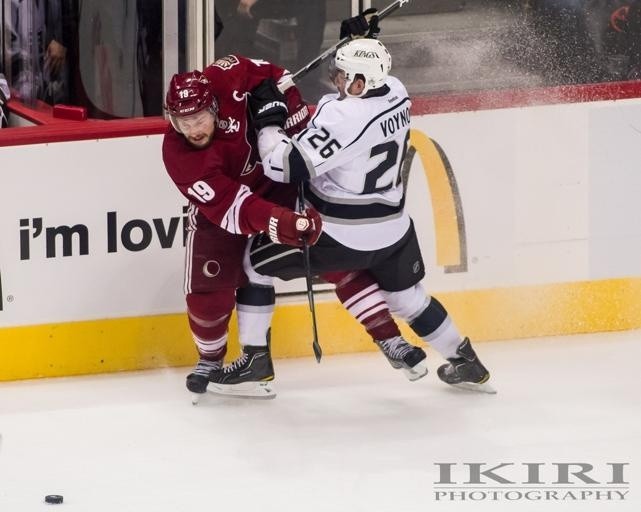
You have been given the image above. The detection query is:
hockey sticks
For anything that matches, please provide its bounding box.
[297,181,322,364]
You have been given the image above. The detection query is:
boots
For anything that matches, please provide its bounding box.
[376,334,427,370]
[436,336,490,385]
[185,326,275,393]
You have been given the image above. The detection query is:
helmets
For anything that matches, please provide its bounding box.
[163,71,214,116]
[327,37,391,98]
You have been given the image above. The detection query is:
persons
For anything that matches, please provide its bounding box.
[43,0,338,120]
[209,8,490,384]
[162,54,427,393]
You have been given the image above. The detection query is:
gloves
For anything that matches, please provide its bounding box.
[266,203,324,250]
[248,77,289,131]
[339,7,380,41]
